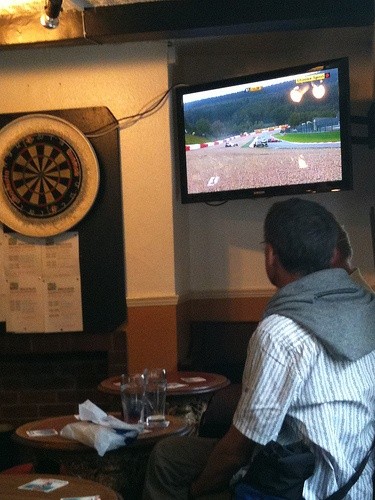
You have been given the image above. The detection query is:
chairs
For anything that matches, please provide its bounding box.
[317,432,375,500]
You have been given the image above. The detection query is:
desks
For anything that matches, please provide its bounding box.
[0,470,125,500]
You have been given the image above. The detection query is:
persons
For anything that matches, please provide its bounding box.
[140,200,375,500]
[329,225,375,300]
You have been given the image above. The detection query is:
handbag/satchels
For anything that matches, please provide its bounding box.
[57,399,145,457]
[229,443,315,500]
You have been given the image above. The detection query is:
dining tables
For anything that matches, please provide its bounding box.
[98,372,230,426]
[15,409,201,486]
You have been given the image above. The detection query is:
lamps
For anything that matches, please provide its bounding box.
[39,0,62,29]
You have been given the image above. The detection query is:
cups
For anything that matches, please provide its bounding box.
[119,373,145,426]
[142,368,168,427]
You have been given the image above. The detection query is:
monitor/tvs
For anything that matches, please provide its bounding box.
[174,56,355,205]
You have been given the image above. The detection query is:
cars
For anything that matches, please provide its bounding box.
[268,138,281,142]
[254,141,268,148]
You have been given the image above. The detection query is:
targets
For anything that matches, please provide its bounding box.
[3,134,82,220]
